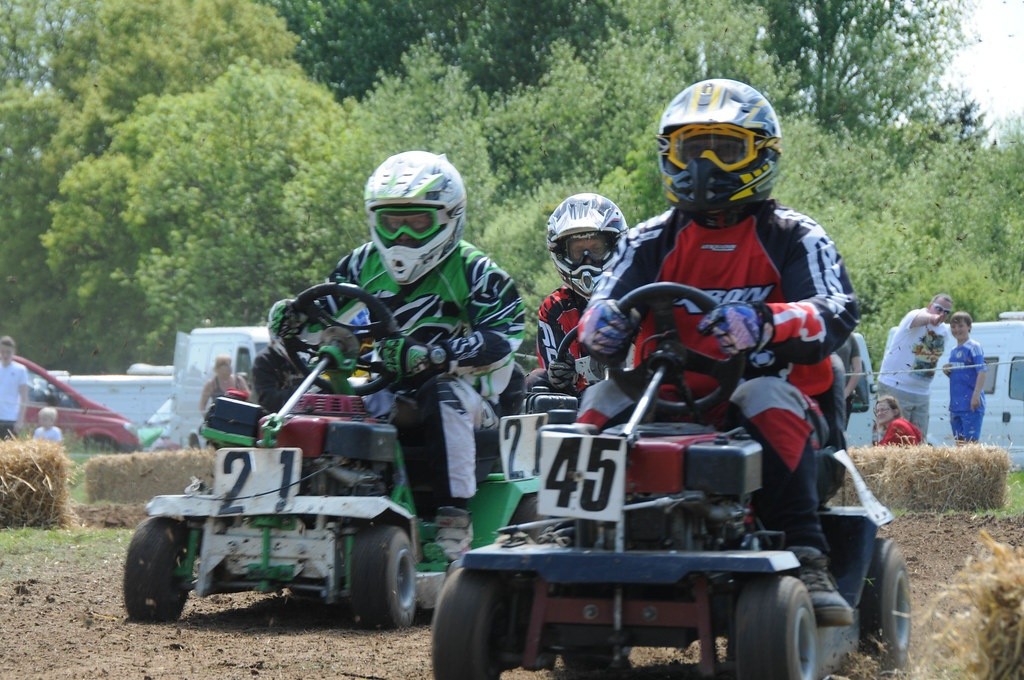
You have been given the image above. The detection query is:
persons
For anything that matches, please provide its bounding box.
[578,75,863,626]
[32,406,63,442]
[877,293,952,438]
[873,396,922,446]
[525,193,631,394]
[942,311,986,442]
[305,150,525,559]
[0,335,29,441]
[249,297,312,413]
[830,333,862,429]
[198,353,251,417]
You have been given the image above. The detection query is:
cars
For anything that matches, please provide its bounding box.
[12,355,150,453]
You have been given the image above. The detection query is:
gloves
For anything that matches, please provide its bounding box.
[547,353,579,396]
[704,298,773,355]
[580,299,641,356]
[385,335,450,386]
[269,298,302,339]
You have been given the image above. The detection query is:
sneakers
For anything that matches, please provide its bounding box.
[435,505,474,561]
[793,553,853,625]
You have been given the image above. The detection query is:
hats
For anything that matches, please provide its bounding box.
[213,355,231,371]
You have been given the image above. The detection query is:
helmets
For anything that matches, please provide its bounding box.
[365,151,467,286]
[546,193,629,297]
[656,78,782,210]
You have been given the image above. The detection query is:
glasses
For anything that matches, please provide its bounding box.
[374,208,441,241]
[667,123,760,170]
[932,303,950,315]
[552,231,616,270]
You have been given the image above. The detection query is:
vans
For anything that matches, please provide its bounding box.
[167,325,272,455]
[888,313,1022,466]
